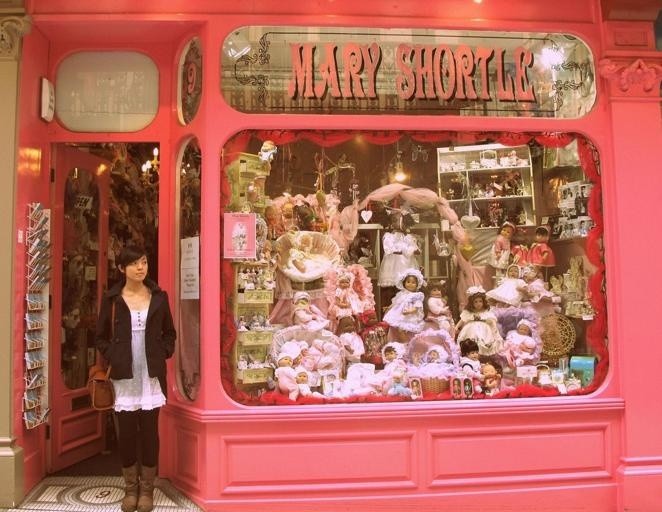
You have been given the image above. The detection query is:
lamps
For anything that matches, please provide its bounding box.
[543,33,577,65]
[392,139,406,181]
[222,30,251,61]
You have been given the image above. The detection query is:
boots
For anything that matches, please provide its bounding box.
[138,464,158,511]
[120,461,139,512]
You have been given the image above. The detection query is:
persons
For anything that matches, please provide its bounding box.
[60,140,598,396]
[96,244,177,512]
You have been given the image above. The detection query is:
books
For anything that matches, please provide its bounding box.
[22,200,53,429]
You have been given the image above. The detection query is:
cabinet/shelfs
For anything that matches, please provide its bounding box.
[437,142,537,235]
[348,222,388,322]
[388,222,448,285]
[541,163,589,243]
[22,202,50,429]
[219,151,275,395]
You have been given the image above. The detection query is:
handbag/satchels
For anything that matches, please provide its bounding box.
[89,366,115,410]
